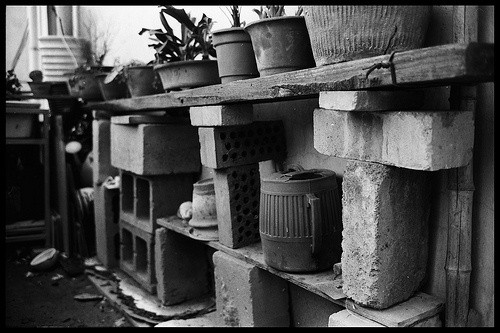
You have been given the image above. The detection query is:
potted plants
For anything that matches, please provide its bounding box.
[105,55,165,97]
[244,5,316,76]
[27,69,52,96]
[209,5,260,83]
[139,4,222,93]
[62,9,114,100]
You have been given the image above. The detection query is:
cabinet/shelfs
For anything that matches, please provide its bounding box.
[5,97,52,251]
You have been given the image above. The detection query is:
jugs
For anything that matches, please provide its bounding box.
[259,160,343,272]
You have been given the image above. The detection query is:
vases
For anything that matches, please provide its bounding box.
[188,177,218,227]
[302,5,430,65]
[95,71,131,100]
